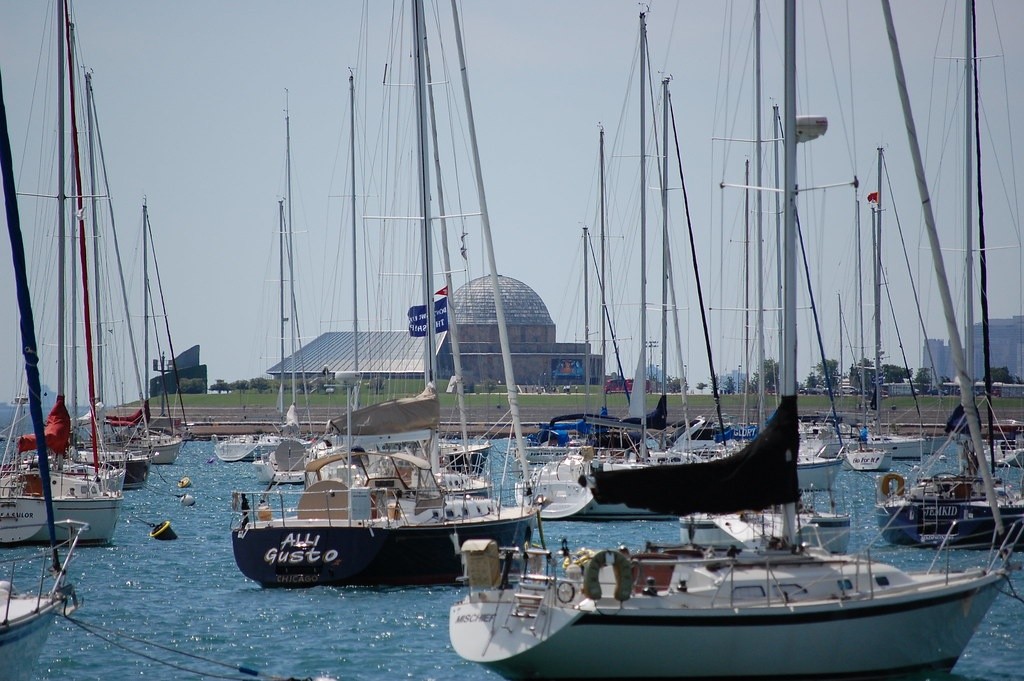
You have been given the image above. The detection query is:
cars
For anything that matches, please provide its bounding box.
[926,387,949,397]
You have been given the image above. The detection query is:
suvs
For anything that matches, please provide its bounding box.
[839,385,859,396]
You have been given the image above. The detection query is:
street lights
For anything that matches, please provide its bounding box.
[644,339,659,380]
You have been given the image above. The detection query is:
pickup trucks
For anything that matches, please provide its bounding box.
[805,384,827,396]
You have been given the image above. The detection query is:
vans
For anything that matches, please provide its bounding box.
[892,386,919,397]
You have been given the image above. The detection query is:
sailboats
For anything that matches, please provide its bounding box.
[2,1,1024,680]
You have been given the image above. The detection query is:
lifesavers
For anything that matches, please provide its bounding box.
[585,549,632,599]
[881,473,905,498]
